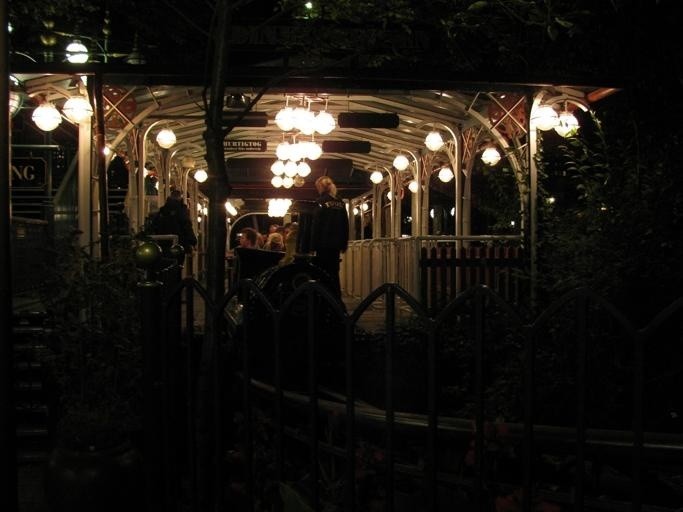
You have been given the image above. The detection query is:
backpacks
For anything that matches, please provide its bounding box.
[154,197,190,228]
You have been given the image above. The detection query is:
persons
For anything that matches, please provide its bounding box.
[225,220,300,290]
[308,176,349,312]
[107,144,199,267]
